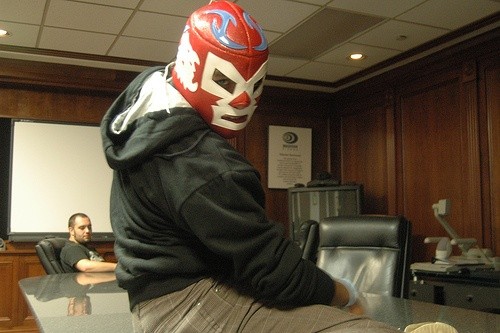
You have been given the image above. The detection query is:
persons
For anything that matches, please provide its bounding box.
[101,0,404,333]
[60,213,116,272]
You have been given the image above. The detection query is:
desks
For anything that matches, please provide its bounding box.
[410,271,500,314]
[19,273,500,333]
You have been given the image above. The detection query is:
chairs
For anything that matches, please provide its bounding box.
[294,215,410,300]
[35,237,69,274]
